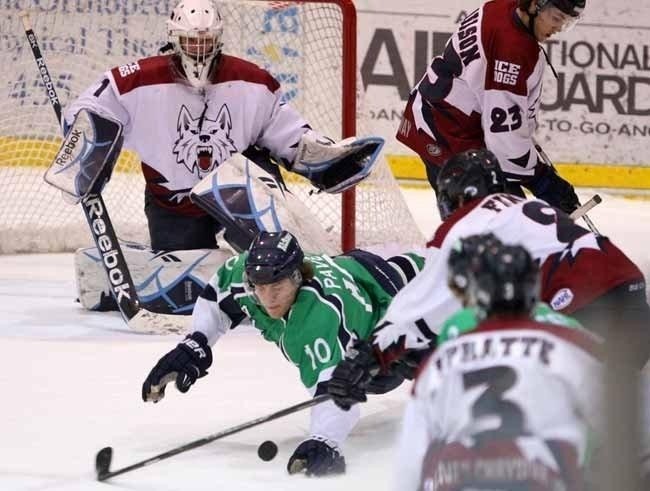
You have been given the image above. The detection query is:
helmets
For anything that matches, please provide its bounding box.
[165,0,224,92]
[436,150,505,219]
[447,234,537,311]
[243,230,304,305]
[538,0,584,32]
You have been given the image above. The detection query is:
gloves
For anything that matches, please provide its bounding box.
[538,167,577,213]
[286,435,345,476]
[327,339,373,411]
[142,332,212,403]
[359,359,420,394]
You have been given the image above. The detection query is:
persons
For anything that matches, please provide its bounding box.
[394,0,586,216]
[315,150,650,412]
[435,232,609,342]
[139,230,423,479]
[53,1,385,313]
[398,249,633,490]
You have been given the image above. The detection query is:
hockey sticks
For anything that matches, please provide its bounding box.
[20,11,194,334]
[95,392,335,481]
